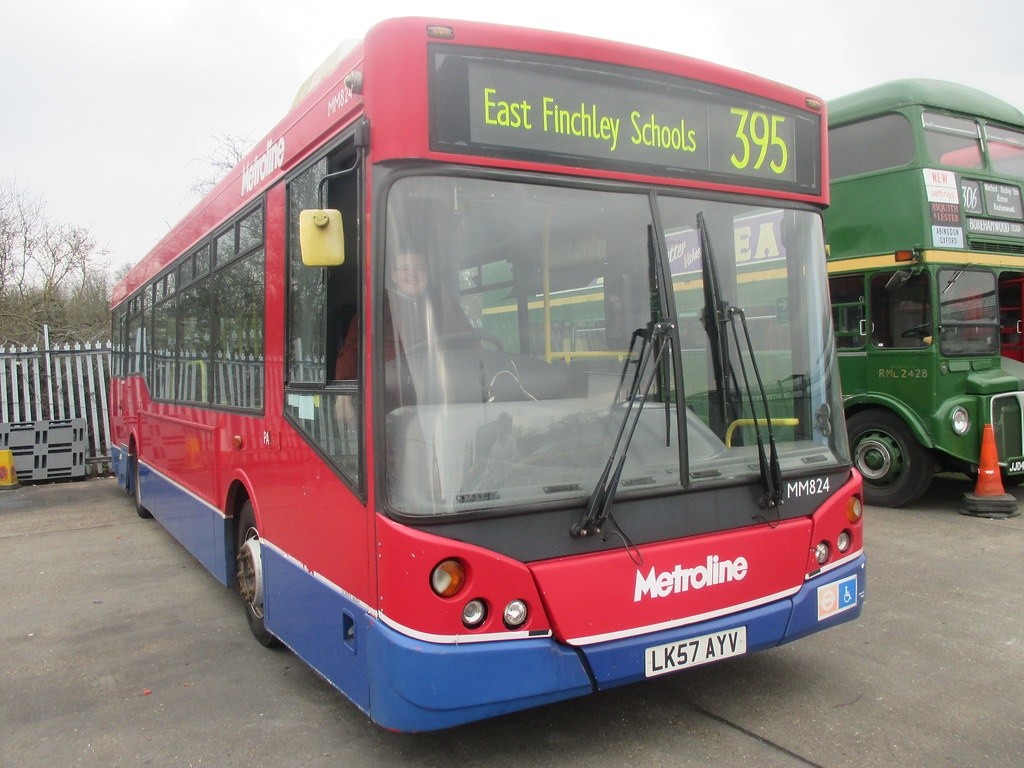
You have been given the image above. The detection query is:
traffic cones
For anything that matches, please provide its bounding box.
[959,424,1018,520]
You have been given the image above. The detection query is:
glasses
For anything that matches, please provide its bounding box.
[393,266,431,274]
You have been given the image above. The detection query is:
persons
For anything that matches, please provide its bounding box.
[333,244,482,442]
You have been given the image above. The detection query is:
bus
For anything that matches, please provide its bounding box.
[474,79,1024,508]
[111,16,873,738]
